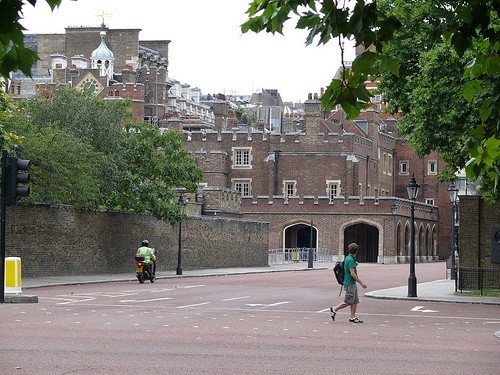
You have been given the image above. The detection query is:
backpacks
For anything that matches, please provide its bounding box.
[333,261,345,286]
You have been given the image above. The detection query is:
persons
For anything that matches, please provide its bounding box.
[137,239,157,275]
[330,243,367,323]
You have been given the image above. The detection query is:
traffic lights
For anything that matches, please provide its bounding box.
[10,156,31,200]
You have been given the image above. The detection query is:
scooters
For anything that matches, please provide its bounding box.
[134,250,160,283]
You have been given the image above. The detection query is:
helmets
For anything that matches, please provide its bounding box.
[141,239,149,246]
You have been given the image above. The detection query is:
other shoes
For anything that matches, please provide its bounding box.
[330,307,336,321]
[349,317,363,324]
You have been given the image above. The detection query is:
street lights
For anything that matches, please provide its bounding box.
[175,194,184,275]
[405,173,421,298]
[447,181,460,279]
